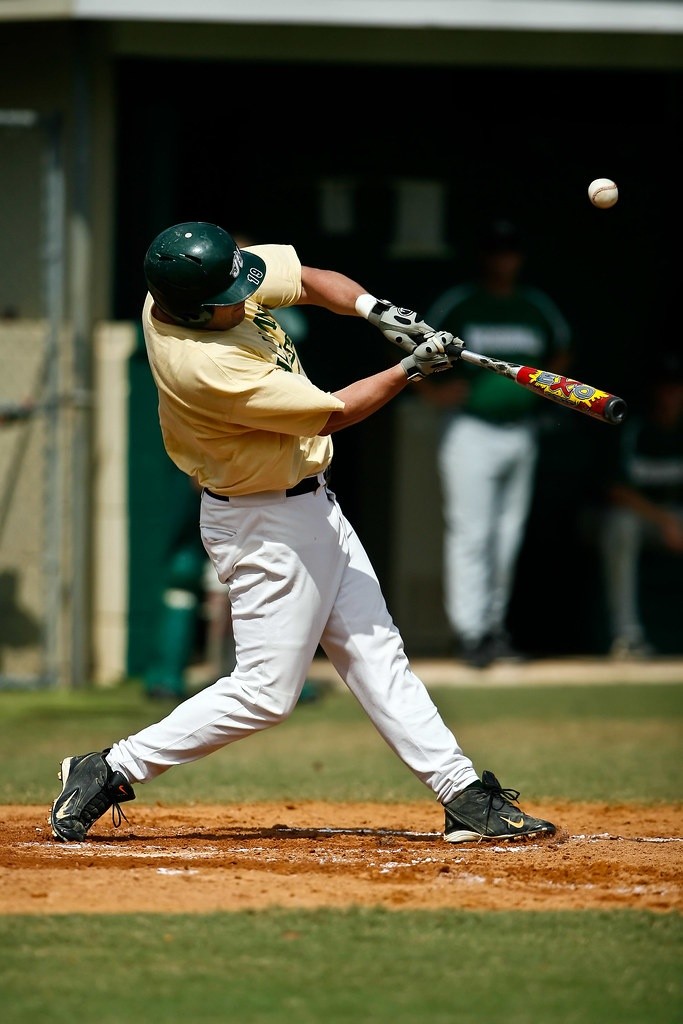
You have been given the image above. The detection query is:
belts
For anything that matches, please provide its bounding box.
[204,471,327,502]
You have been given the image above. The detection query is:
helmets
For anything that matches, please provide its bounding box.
[144,222,266,325]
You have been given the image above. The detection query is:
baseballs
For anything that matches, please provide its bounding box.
[587,177,619,209]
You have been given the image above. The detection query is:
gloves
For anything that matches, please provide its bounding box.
[368,299,437,355]
[400,331,467,384]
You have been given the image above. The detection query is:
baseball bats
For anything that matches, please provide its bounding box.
[420,332,629,426]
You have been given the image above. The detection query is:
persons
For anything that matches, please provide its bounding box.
[596,373,683,656]
[181,232,308,695]
[49,220,557,844]
[385,218,576,655]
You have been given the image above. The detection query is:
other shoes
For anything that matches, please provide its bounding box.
[609,636,657,661]
[463,632,533,668]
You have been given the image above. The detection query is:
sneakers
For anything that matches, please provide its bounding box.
[47,747,136,843]
[443,770,556,844]
